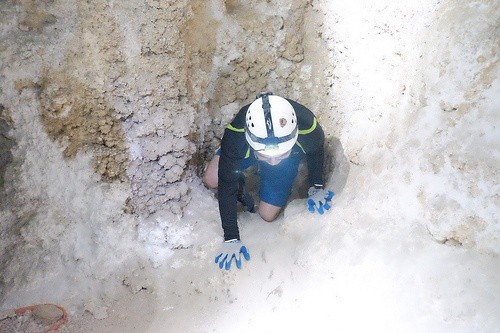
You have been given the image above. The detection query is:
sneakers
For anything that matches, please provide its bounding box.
[238,173,258,214]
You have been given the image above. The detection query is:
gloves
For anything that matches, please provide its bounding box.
[307,187,334,214]
[215,239,249,270]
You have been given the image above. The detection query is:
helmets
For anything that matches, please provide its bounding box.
[244,92,299,157]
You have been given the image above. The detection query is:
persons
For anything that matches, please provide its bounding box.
[202,91,337,271]
